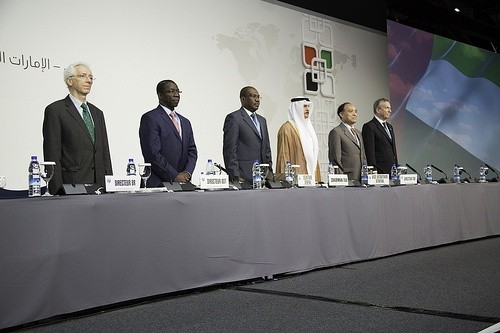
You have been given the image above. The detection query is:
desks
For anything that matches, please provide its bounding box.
[0,180,500,332]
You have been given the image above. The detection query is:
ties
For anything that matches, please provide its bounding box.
[250,113,257,125]
[382,122,390,137]
[350,128,358,142]
[169,112,180,135]
[80,103,95,144]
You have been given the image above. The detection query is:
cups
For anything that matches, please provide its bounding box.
[397,166,408,177]
[0,176,6,189]
[423,168,429,176]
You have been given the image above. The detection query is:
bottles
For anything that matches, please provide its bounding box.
[361,163,368,187]
[252,160,262,189]
[453,164,460,183]
[206,159,215,191]
[479,165,486,183]
[284,160,294,189]
[427,165,433,181]
[28,155,41,197]
[391,163,398,182]
[327,162,335,187]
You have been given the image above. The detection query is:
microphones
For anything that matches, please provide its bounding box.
[431,165,455,183]
[454,164,480,183]
[151,161,182,191]
[333,159,355,187]
[405,164,422,180]
[55,159,88,195]
[265,166,282,189]
[373,163,394,186]
[484,163,500,182]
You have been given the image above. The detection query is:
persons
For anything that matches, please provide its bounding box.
[223,86,274,184]
[276,96,321,184]
[43,64,113,194]
[361,98,398,179]
[329,102,367,182]
[139,80,198,188]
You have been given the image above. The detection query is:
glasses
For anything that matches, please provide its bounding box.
[160,89,182,96]
[68,74,94,81]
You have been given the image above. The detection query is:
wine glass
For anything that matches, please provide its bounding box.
[126,158,136,176]
[214,164,221,191]
[258,166,269,190]
[39,164,54,197]
[291,165,300,190]
[138,164,152,193]
